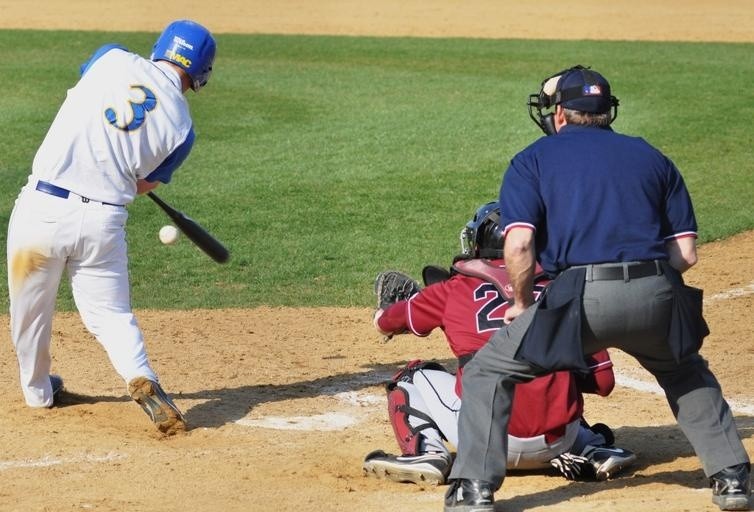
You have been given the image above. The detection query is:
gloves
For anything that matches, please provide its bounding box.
[549,452,597,483]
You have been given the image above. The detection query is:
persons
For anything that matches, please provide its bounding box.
[443,66,754,512]
[6,20,217,436]
[363,201,636,486]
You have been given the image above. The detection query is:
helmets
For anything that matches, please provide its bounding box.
[150,20,216,93]
[527,65,620,136]
[459,201,504,259]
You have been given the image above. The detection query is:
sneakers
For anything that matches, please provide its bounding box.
[362,450,450,485]
[128,376,187,436]
[443,478,495,512]
[710,463,751,511]
[49,375,63,395]
[582,444,637,482]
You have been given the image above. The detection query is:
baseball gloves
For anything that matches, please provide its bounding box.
[374,271,423,344]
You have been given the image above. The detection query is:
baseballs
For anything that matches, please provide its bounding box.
[159,226,179,246]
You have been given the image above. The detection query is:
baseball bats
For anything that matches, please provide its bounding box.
[147,191,229,264]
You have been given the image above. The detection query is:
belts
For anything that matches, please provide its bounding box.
[36,180,125,207]
[545,425,565,443]
[584,262,664,281]
